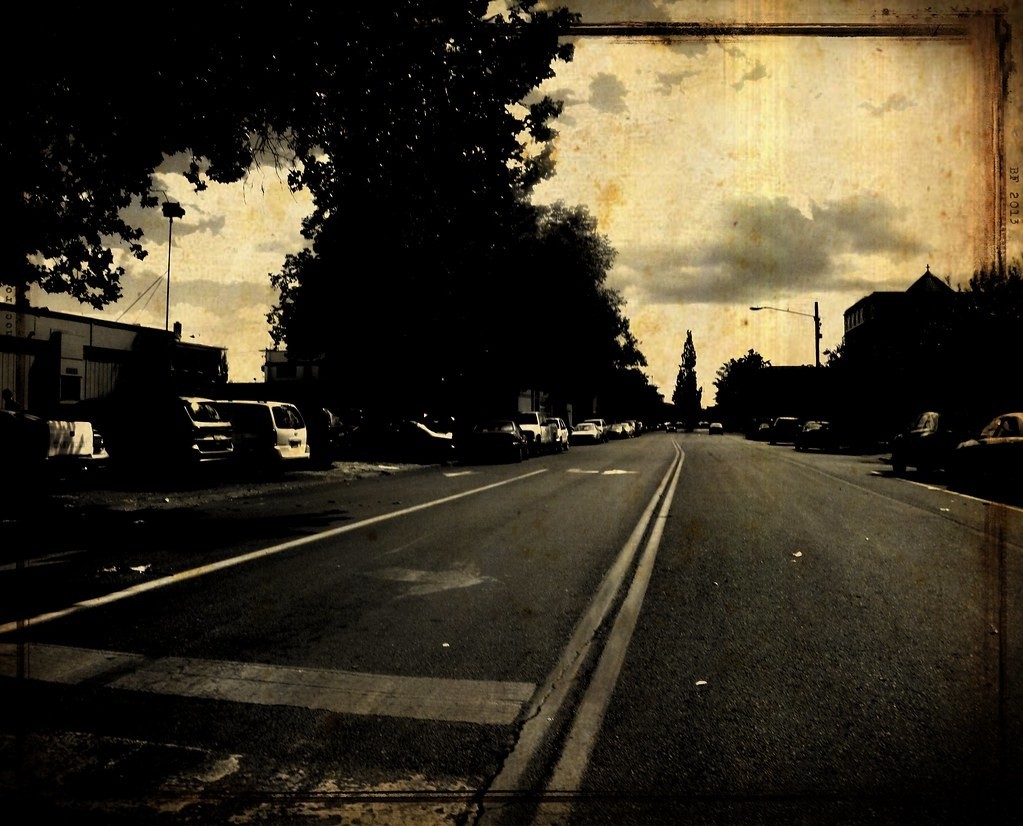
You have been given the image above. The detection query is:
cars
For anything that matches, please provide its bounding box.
[769,416,844,452]
[1,412,113,497]
[884,409,1023,496]
[306,405,665,460]
[665,424,677,434]
[708,421,725,436]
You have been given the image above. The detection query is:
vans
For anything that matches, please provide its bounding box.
[95,396,310,487]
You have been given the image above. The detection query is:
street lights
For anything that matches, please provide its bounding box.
[748,300,822,367]
